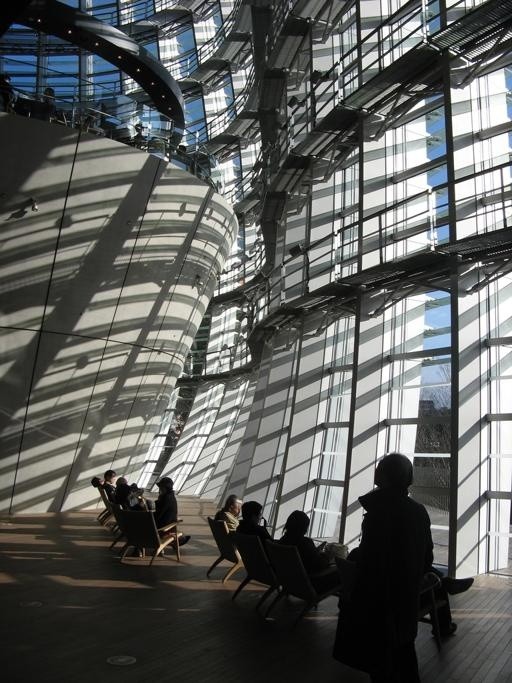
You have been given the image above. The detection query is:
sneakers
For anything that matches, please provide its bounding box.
[131,549,143,558]
[431,622,457,636]
[449,578,474,595]
[172,535,191,549]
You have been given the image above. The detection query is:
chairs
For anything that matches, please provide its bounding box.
[205,516,445,652]
[96,476,183,567]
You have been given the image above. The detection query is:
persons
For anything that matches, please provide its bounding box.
[347,545,474,636]
[331,452,435,683]
[234,500,275,540]
[102,469,119,504]
[114,476,131,504]
[214,493,244,532]
[124,485,191,548]
[150,476,178,535]
[276,509,345,597]
[91,476,106,488]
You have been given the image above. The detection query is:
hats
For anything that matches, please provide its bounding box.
[156,477,174,490]
